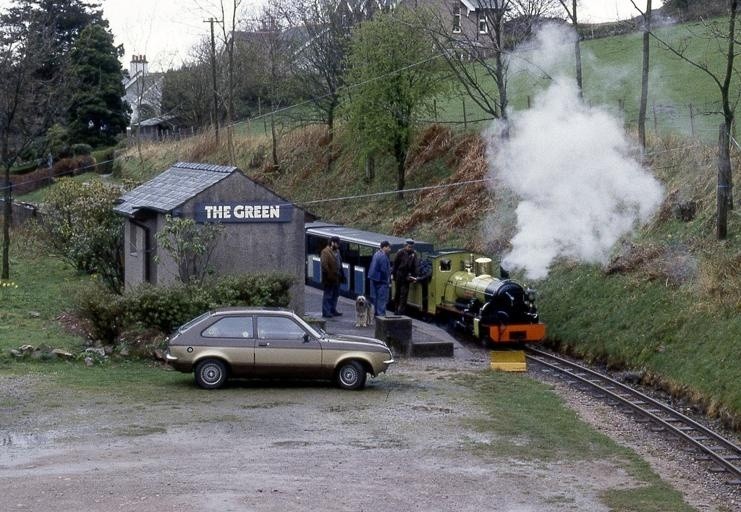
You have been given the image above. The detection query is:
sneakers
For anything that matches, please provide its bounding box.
[323,311,343,317]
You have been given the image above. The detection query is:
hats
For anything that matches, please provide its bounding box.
[407,239,414,244]
[330,236,340,243]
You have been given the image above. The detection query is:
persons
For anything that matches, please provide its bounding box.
[320,237,347,318]
[393,240,418,315]
[368,240,391,318]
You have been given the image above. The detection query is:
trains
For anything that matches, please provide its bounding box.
[305,218,545,346]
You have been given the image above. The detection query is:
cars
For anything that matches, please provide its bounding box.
[157,305,394,388]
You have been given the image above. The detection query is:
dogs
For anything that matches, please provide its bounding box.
[354,294,375,328]
